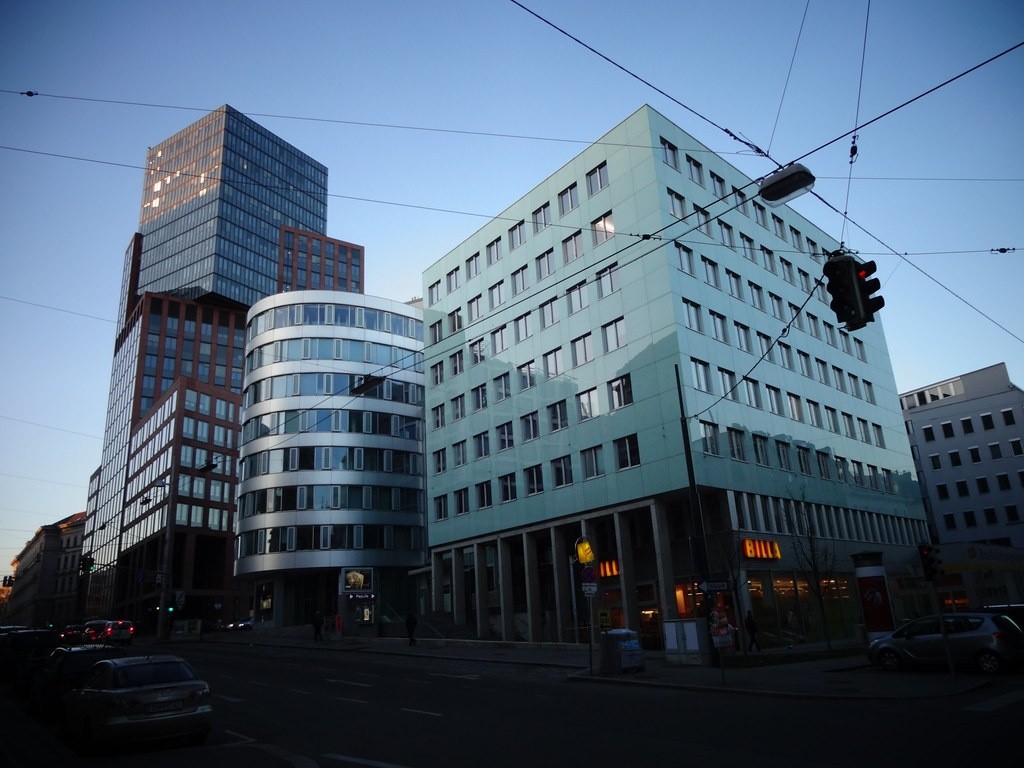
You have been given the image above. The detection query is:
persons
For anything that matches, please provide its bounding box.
[709,611,719,635]
[782,607,805,647]
[405,612,417,647]
[310,610,324,642]
[745,610,759,652]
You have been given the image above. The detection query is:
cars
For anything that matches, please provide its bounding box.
[225,617,254,631]
[30,643,126,731]
[956,602,1024,631]
[67,654,214,754]
[58,624,83,641]
[82,620,109,642]
[101,619,133,645]
[0,624,58,694]
[866,612,1024,675]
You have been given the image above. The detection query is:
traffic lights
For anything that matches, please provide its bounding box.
[917,544,938,578]
[822,253,863,331]
[167,595,176,613]
[853,259,885,326]
[81,559,88,573]
[86,558,95,571]
